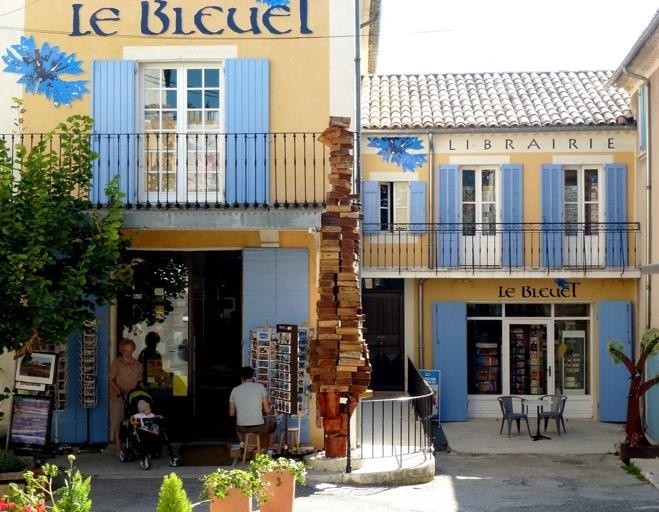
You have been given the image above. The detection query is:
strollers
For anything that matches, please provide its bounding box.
[115,390,181,469]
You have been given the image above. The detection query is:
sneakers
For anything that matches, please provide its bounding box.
[239,442,246,455]
[267,448,274,457]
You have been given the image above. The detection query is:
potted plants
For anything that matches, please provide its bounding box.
[249,450,313,511]
[199,468,271,512]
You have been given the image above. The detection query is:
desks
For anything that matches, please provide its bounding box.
[522,400,553,442]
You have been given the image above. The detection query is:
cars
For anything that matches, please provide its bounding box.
[366,322,403,358]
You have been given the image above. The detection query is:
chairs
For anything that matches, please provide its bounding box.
[497,396,532,439]
[537,395,568,436]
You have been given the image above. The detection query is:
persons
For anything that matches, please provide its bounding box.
[138,332,161,392]
[108,338,148,457]
[228,366,277,461]
[130,400,164,439]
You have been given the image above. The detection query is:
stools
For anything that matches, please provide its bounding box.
[279,427,300,454]
[242,433,261,466]
